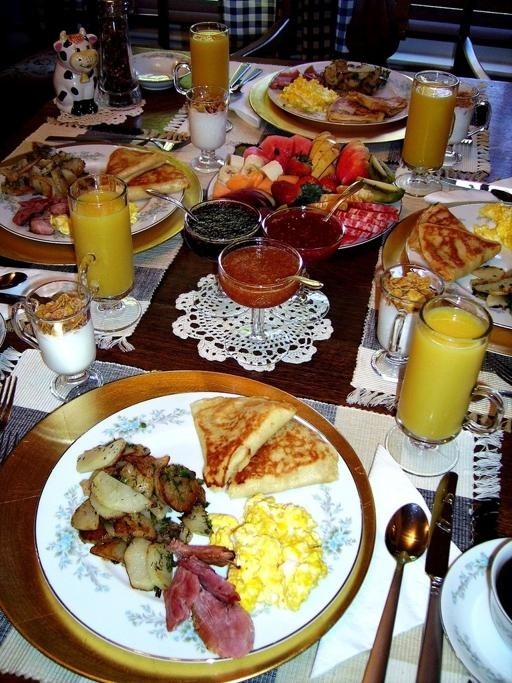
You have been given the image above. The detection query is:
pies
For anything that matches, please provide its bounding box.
[328,91,408,124]
[192,395,339,500]
[408,201,501,281]
[107,148,190,201]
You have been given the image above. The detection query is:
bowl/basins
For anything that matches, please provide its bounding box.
[128,49,191,91]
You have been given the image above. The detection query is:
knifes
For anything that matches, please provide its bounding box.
[436,173,512,199]
[415,468,461,681]
[1,270,29,308]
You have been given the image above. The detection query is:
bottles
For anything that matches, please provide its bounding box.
[94,1,141,109]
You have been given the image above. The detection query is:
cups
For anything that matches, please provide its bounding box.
[486,538,512,646]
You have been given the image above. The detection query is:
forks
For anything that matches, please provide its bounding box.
[0,373,20,466]
[41,135,150,147]
[76,130,193,150]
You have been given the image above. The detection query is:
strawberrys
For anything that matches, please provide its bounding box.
[271,174,340,206]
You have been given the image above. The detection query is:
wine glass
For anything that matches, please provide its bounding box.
[183,197,344,366]
[67,173,143,332]
[10,276,103,403]
[172,19,234,172]
[371,263,446,382]
[394,66,492,198]
[385,295,504,479]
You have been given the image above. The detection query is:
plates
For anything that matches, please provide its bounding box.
[206,159,402,250]
[439,533,511,683]
[34,392,363,666]
[267,57,417,127]
[0,142,188,245]
[403,200,511,330]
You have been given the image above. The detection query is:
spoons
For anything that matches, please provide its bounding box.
[362,502,432,683]
[228,67,264,102]
